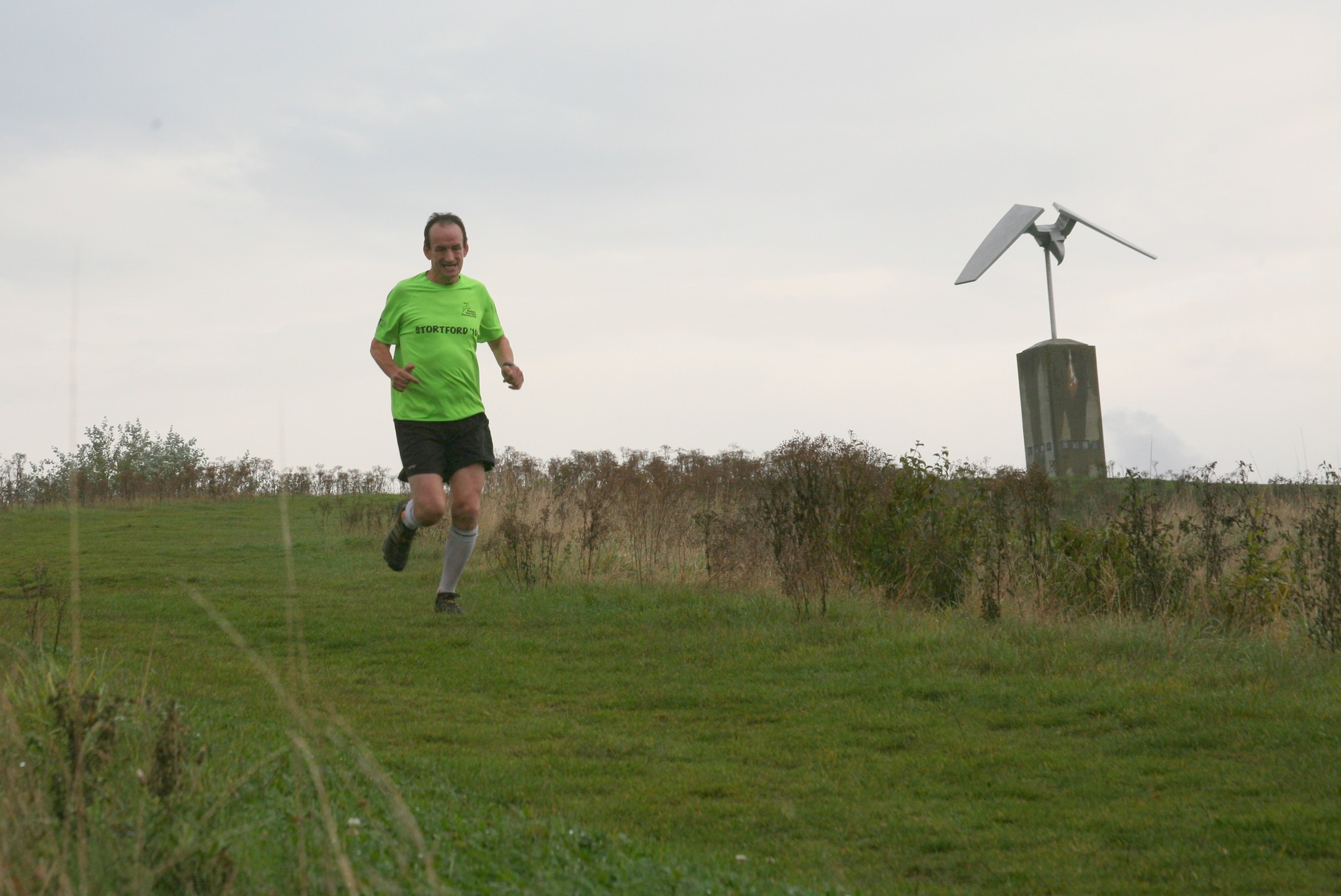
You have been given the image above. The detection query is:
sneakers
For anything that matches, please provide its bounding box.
[434,591,462,614]
[382,501,417,572]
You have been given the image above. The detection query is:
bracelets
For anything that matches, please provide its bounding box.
[501,362,515,366]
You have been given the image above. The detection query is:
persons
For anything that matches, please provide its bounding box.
[370,212,525,616]
